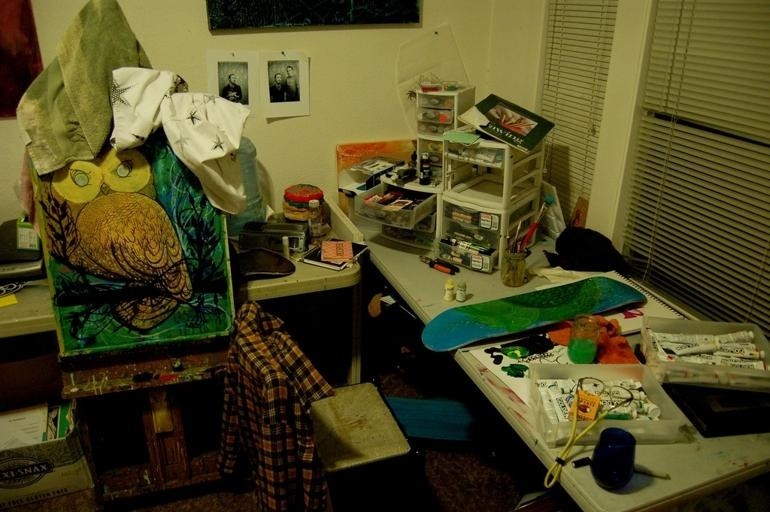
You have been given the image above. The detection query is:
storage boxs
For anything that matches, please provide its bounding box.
[0,396,94,507]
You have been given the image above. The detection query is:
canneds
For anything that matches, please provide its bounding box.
[283,184,324,245]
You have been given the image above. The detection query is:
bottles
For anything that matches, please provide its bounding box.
[419,152,431,185]
[444,278,454,301]
[456,279,467,302]
[308,200,322,245]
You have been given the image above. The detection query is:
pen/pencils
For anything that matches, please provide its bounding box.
[419,255,460,275]
[440,236,496,268]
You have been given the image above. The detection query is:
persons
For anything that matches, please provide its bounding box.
[285,66,300,102]
[269,73,286,103]
[221,74,242,102]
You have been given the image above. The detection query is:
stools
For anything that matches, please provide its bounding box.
[310,383,410,510]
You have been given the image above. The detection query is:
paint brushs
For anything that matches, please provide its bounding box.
[506,194,554,253]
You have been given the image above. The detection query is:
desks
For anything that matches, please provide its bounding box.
[359,223,751,511]
[1,250,362,387]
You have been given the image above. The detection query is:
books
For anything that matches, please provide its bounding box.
[301,237,369,271]
[456,94,555,154]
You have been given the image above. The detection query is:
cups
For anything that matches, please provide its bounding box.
[501,250,527,287]
[590,427,637,490]
[567,313,600,364]
